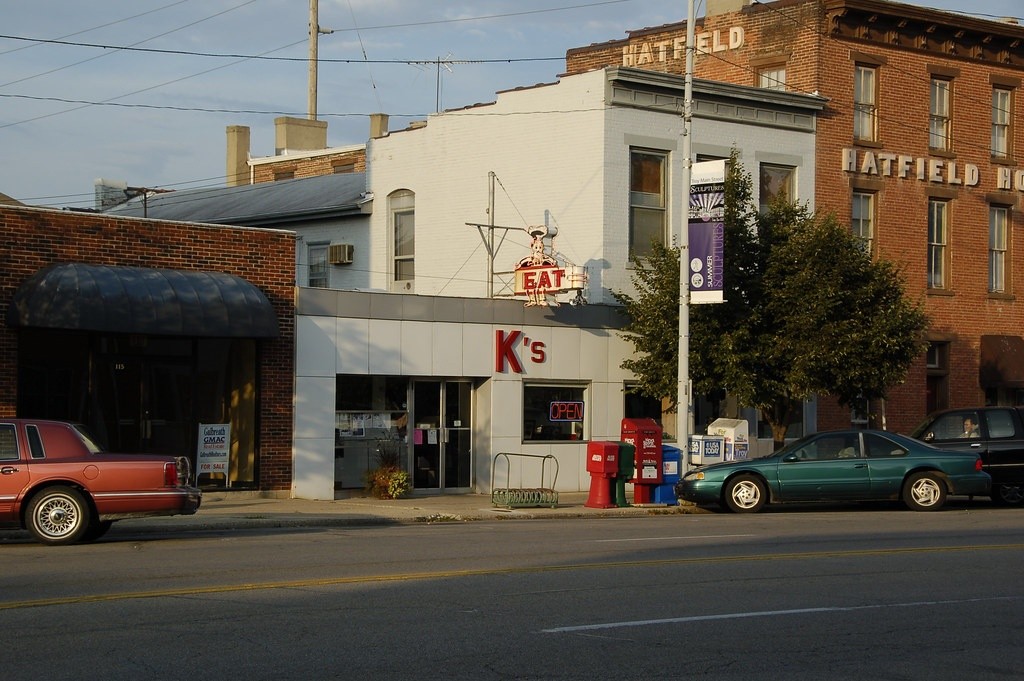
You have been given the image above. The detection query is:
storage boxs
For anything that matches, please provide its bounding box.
[688,434,724,464]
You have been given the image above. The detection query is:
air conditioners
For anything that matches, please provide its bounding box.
[327,243,354,264]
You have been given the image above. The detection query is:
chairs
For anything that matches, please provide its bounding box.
[837,446,855,459]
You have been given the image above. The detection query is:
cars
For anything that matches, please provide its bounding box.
[671,428,993,515]
[0,417,203,546]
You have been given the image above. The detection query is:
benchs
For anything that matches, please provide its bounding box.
[890,449,905,455]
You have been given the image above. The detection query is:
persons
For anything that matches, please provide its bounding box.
[956,417,981,438]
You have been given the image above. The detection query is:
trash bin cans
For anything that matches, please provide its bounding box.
[654,445,682,506]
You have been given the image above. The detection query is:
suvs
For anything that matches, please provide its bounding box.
[892,406,1024,509]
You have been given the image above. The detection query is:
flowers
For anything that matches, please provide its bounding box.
[364,432,412,499]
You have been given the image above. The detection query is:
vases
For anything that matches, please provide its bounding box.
[379,486,392,500]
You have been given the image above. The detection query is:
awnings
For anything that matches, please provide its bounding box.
[15,261,280,340]
